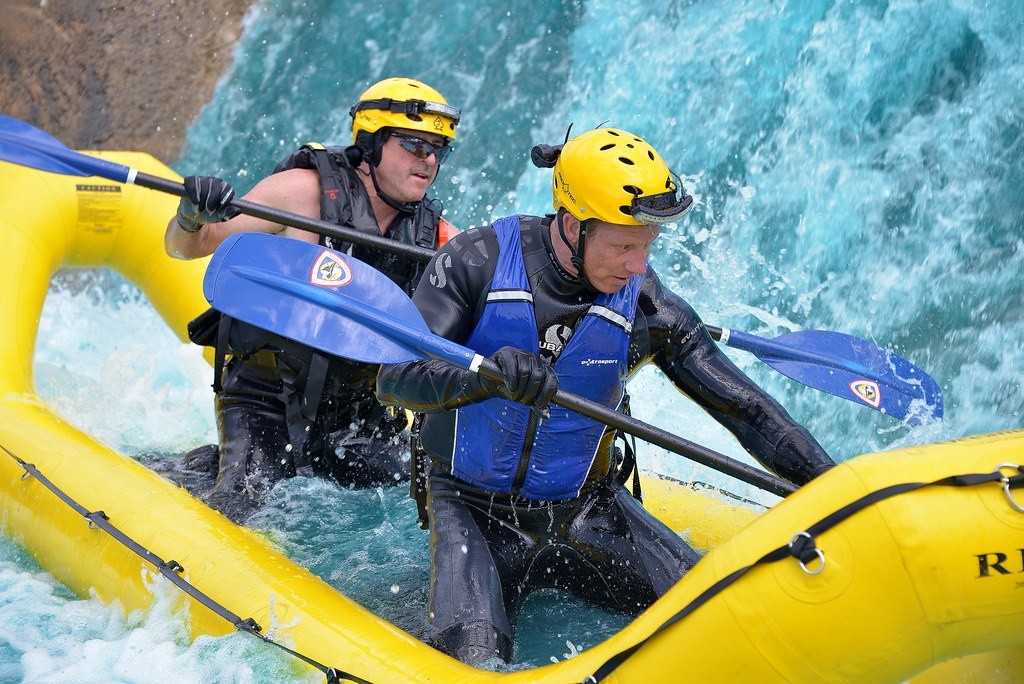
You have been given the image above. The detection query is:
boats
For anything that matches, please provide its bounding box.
[1,114,1024,684]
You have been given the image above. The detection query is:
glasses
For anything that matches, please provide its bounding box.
[390,132,454,165]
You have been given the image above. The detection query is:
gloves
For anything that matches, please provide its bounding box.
[488,345,559,419]
[176,175,242,233]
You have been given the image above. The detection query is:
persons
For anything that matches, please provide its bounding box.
[375,127,836,672]
[165,78,464,493]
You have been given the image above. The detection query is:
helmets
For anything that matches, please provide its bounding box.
[349,77,456,144]
[552,127,677,226]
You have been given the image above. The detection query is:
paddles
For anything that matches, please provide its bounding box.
[0,113,945,425]
[201,231,800,499]
